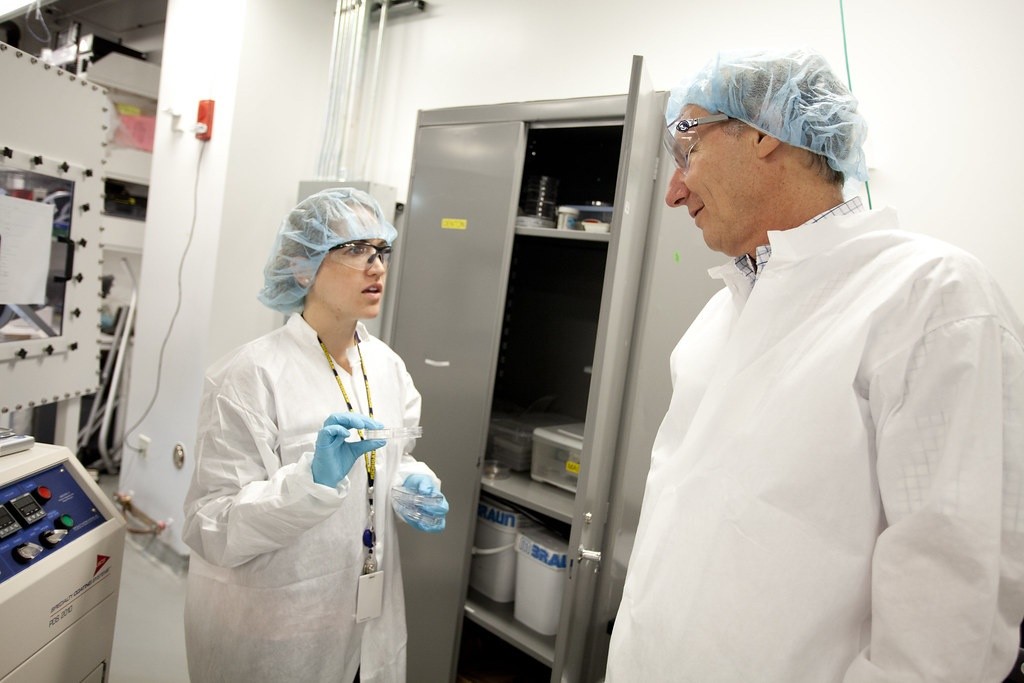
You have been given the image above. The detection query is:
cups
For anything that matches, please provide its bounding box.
[525,176,559,221]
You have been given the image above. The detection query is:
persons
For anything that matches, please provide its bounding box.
[604,45,1024,683]
[183,186,450,683]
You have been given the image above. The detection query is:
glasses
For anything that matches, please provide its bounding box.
[307,240,393,272]
[662,108,755,175]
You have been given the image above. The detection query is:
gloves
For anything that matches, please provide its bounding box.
[310,412,387,489]
[398,474,449,533]
